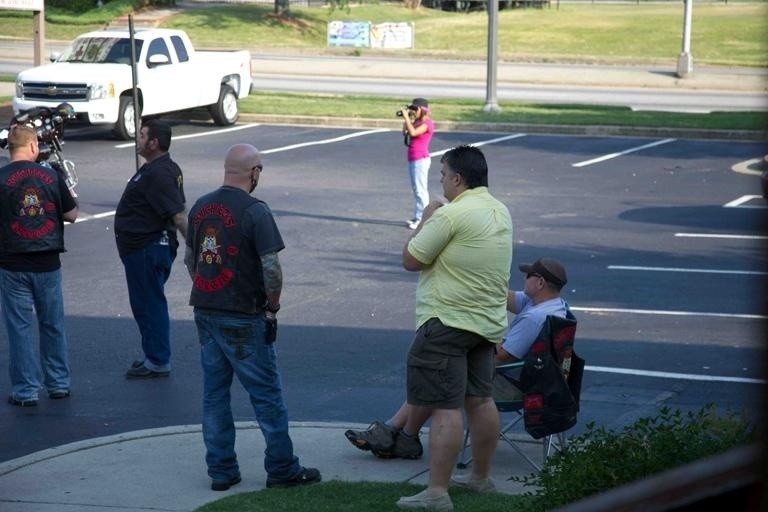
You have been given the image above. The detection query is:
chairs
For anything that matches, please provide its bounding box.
[454,306,587,488]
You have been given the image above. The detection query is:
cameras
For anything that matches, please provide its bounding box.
[396,109,414,117]
[0,103,74,162]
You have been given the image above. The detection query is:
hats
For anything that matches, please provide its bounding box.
[408,98,429,109]
[519,258,567,289]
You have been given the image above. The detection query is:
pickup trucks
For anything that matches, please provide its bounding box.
[14,31,251,138]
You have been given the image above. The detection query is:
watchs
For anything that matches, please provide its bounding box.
[265,304,280,313]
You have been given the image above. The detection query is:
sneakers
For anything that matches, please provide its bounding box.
[345,420,398,459]
[392,431,423,460]
[397,491,453,512]
[450,473,497,493]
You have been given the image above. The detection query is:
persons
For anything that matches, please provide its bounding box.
[398,99,434,231]
[182,144,322,489]
[345,259,568,461]
[0,126,78,407]
[114,123,188,380]
[397,147,514,507]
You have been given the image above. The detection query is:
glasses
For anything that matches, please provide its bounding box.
[252,165,263,172]
[526,271,542,279]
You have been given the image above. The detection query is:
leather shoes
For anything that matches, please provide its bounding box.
[8,393,39,406]
[266,467,322,489]
[127,364,170,379]
[132,361,146,368]
[50,391,72,399]
[212,477,242,491]
